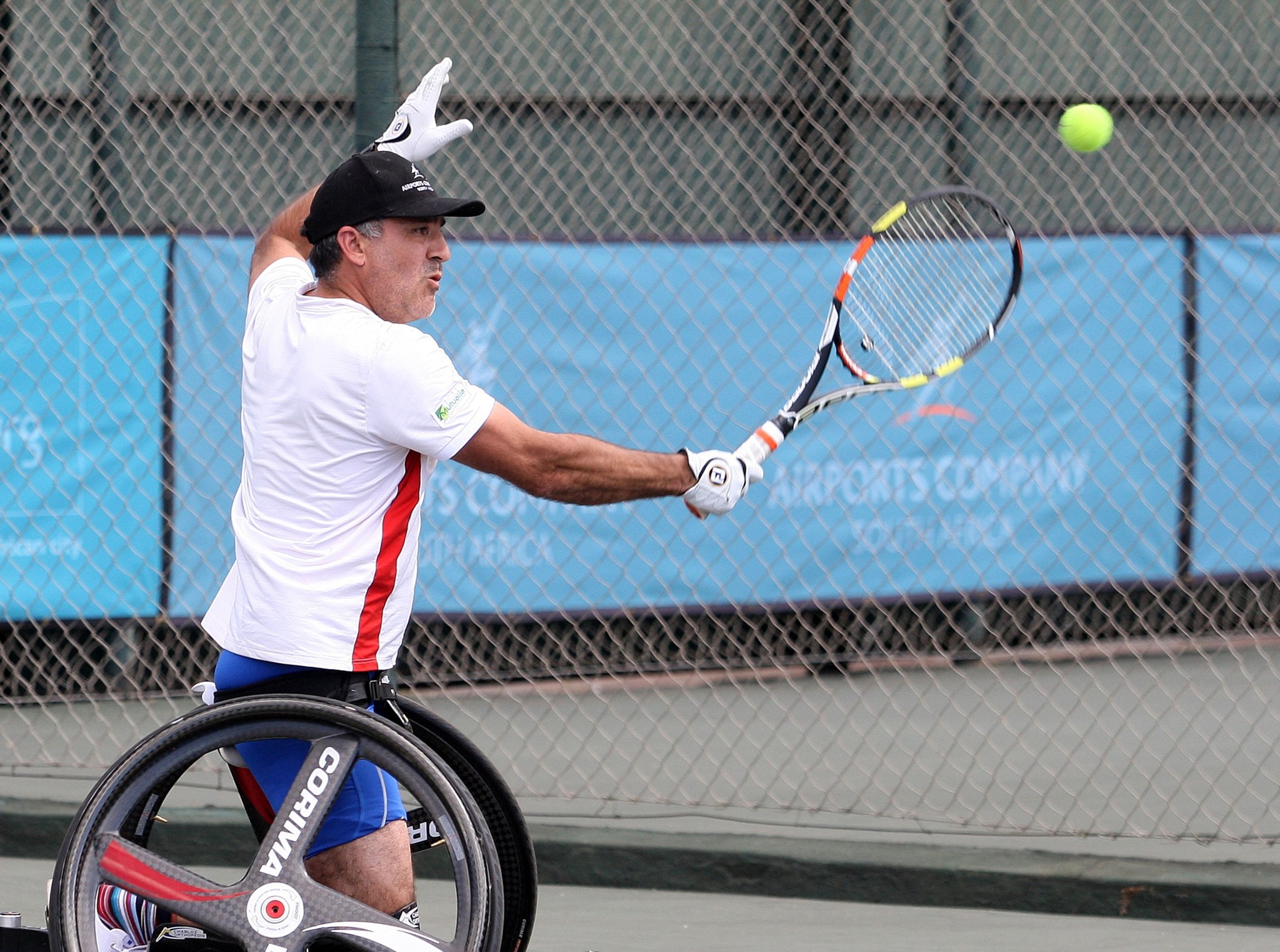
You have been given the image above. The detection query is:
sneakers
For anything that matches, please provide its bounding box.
[44,877,132,952]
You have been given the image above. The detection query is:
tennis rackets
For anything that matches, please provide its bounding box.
[686,185,1023,519]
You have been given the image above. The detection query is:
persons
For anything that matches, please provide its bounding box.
[200,57,764,952]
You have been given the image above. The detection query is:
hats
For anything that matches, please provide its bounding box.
[303,150,486,243]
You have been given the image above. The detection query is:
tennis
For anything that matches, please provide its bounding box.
[1059,104,1112,153]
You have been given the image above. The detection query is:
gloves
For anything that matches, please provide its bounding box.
[676,447,764,514]
[374,57,475,161]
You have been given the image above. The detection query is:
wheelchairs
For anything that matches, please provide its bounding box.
[0,675,538,952]
